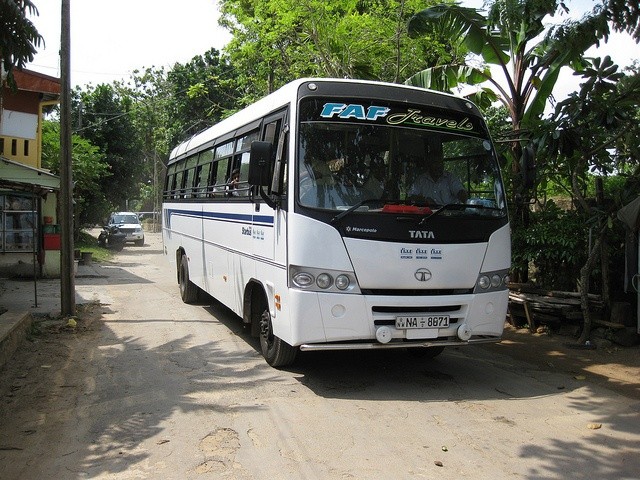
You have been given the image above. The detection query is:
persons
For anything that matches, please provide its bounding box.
[306,151,334,184]
[230,169,251,196]
[363,157,388,202]
[406,150,468,205]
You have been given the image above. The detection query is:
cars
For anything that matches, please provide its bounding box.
[108,212,144,246]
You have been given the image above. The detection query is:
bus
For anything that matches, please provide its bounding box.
[162,78,510,369]
